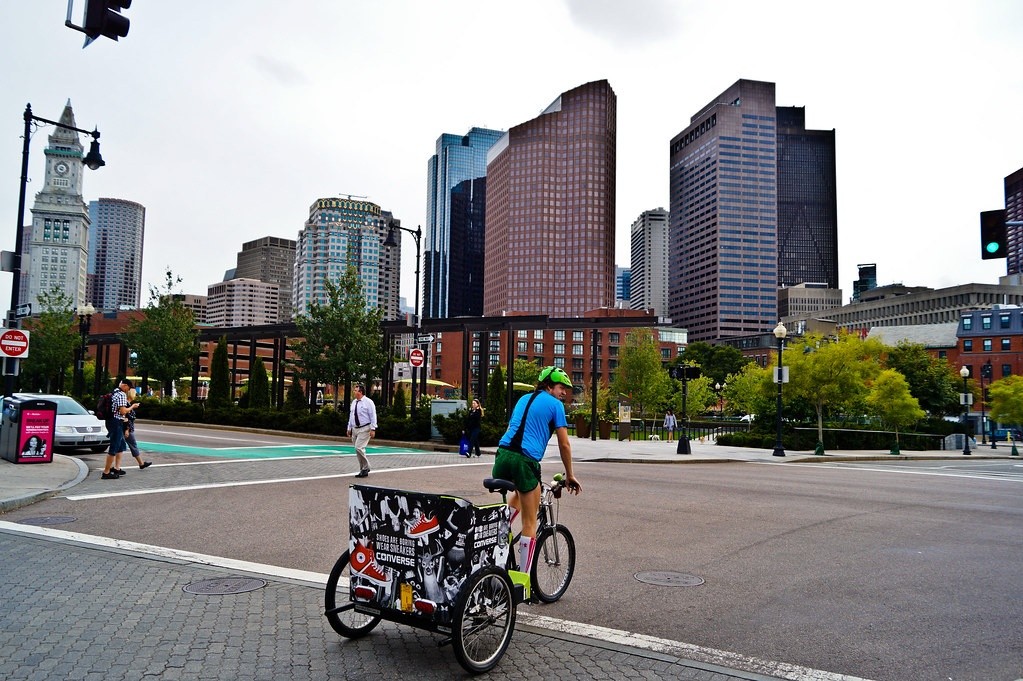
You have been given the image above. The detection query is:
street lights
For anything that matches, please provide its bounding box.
[959,365,973,456]
[773,320,788,457]
[2,102,107,411]
[381,217,423,418]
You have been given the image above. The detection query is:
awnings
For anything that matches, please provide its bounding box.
[961,312,1010,317]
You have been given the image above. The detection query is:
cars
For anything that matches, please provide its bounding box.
[5,392,111,452]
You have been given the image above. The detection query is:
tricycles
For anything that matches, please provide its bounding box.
[320,470,582,677]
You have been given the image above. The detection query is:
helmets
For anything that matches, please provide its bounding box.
[536,364,573,389]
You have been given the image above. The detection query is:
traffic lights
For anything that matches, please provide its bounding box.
[979,209,1009,260]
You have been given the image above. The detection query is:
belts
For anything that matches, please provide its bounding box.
[355,422,371,428]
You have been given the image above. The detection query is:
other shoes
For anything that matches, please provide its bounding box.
[100,472,120,479]
[109,467,127,475]
[473,454,482,457]
[139,461,152,469]
[355,469,370,477]
[465,451,470,458]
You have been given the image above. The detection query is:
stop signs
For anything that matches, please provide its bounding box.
[409,348,425,368]
[0,328,30,358]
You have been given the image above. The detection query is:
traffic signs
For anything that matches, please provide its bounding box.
[15,302,33,320]
[417,334,436,344]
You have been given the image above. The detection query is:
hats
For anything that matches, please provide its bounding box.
[121,377,135,389]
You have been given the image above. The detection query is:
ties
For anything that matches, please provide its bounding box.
[354,400,361,427]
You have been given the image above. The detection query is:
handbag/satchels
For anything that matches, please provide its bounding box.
[460,439,469,455]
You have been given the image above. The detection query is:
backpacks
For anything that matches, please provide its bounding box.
[95,387,123,420]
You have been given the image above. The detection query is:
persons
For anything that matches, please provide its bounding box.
[663,408,677,443]
[317,390,323,400]
[492,366,583,604]
[116,386,152,469]
[101,378,138,479]
[347,385,378,478]
[462,399,484,458]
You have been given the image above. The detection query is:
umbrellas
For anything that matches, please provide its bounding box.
[393,378,455,388]
[487,381,536,392]
[239,375,293,383]
[109,376,211,395]
